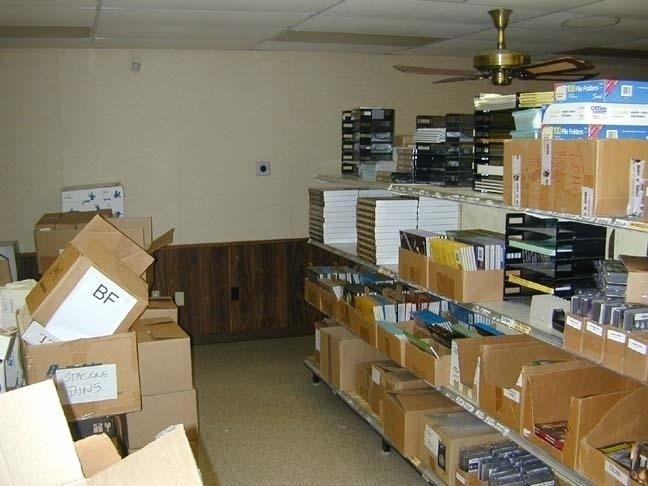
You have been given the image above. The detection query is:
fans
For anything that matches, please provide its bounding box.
[392,10,600,85]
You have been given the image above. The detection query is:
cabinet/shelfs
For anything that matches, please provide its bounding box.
[303,175,648,486]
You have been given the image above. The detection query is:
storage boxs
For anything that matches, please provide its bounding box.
[304,248,648,485]
[0,182,205,486]
[502,139,648,217]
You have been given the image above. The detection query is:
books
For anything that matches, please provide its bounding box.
[567,258,647,331]
[507,234,573,256]
[412,126,445,143]
[508,273,570,294]
[359,146,412,183]
[591,441,647,484]
[471,91,515,193]
[534,418,567,451]
[307,186,506,358]
[517,90,554,106]
[456,439,555,485]
